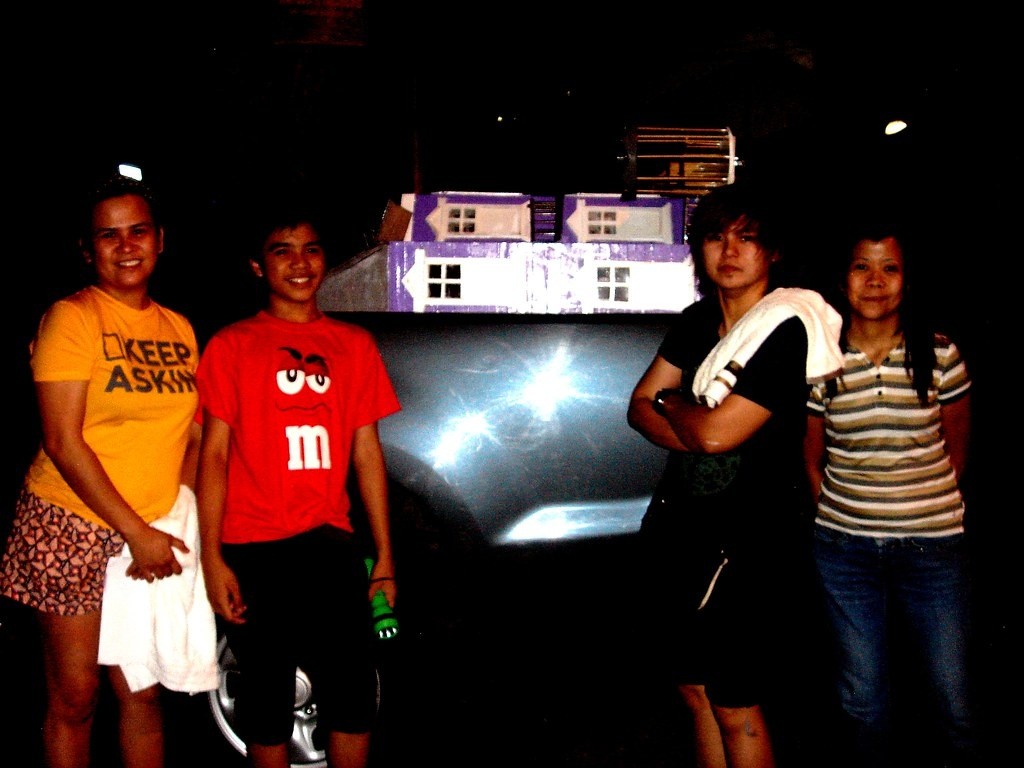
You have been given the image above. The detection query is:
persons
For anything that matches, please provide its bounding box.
[626,181,977,768]
[0,175,403,768]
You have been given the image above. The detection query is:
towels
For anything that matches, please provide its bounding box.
[96,484,222,694]
[689,285,846,411]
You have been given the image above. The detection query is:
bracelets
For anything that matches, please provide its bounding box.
[653,388,671,414]
[369,578,394,582]
